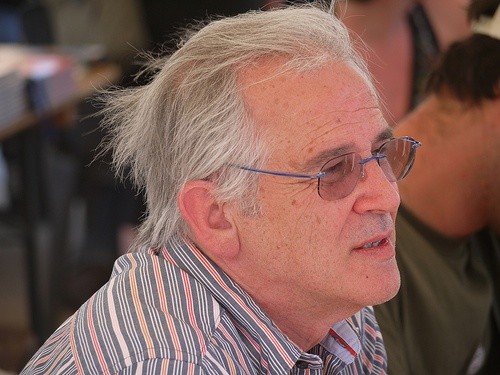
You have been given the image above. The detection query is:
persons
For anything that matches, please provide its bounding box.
[373,0,500,375]
[18,4,402,375]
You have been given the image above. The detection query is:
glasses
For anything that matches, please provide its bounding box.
[222,136,421,202]
